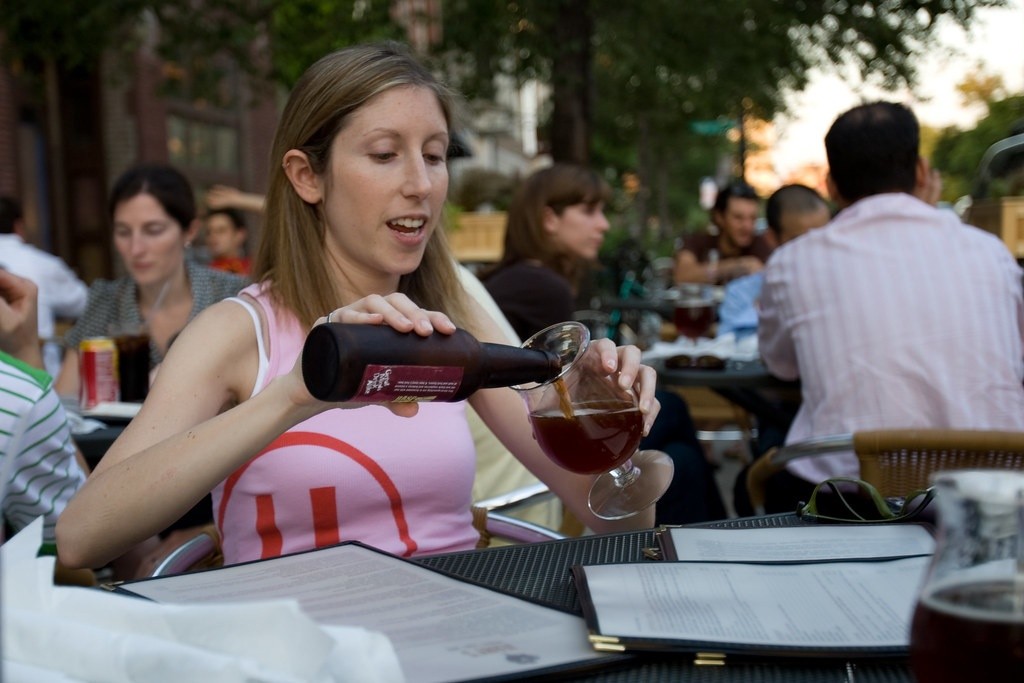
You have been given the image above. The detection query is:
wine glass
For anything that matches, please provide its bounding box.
[674,299,714,370]
[505,320,674,520]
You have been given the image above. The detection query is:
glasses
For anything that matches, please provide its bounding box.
[795,477,980,536]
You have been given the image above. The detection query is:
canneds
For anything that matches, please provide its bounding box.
[79,336,121,411]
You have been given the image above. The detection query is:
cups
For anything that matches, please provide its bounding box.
[911,469,1024,682]
[108,322,151,404]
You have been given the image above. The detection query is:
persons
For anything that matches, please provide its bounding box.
[0,43,1024,586]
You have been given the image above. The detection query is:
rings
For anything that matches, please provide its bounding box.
[327,313,334,323]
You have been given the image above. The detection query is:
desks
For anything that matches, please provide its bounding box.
[66,407,214,531]
[641,357,805,465]
[399,495,933,683]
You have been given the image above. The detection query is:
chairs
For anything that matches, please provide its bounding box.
[676,378,1024,512]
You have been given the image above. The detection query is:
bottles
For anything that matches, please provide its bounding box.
[302,321,562,402]
